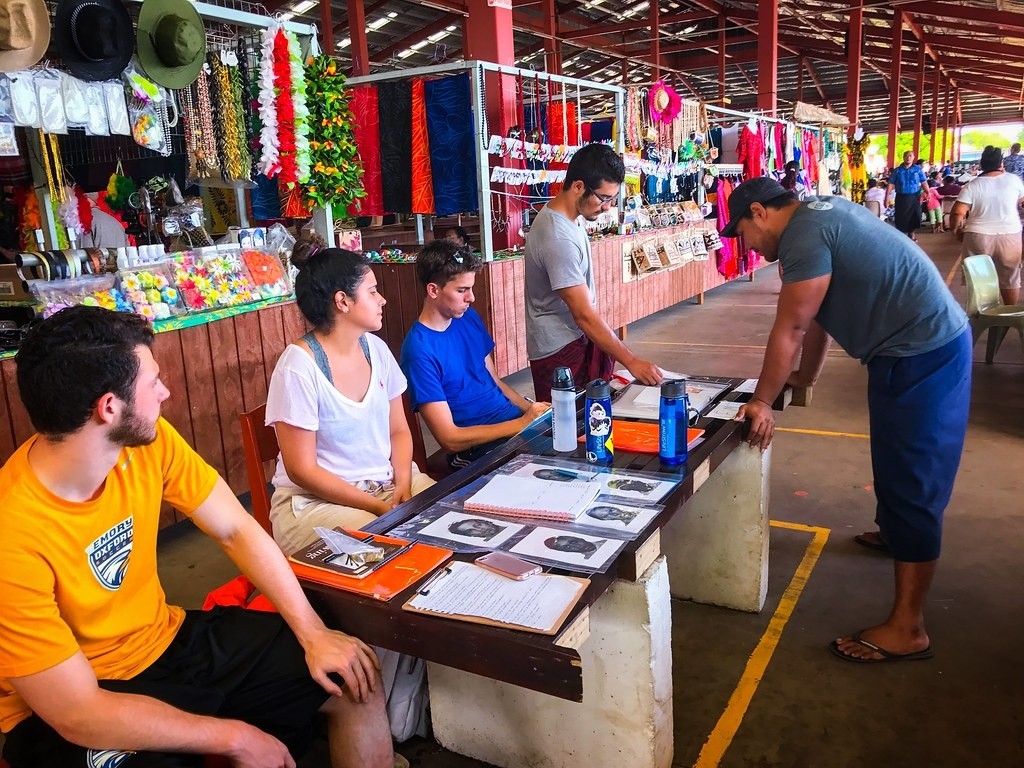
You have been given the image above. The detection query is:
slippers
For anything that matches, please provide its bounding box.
[853,531,892,553]
[829,629,935,663]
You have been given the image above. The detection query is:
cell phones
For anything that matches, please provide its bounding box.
[475,551,543,581]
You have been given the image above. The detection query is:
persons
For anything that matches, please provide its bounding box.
[586,507,641,526]
[75,206,130,249]
[1003,143,1024,186]
[265,237,437,556]
[864,158,983,233]
[718,177,974,660]
[450,519,506,542]
[446,227,481,253]
[885,151,930,240]
[0,303,395,768]
[400,240,552,483]
[608,479,662,497]
[544,535,607,560]
[950,146,1024,306]
[524,144,663,402]
[534,468,575,482]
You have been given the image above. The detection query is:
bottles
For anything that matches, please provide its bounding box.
[584,378,615,468]
[550,366,577,452]
[657,378,691,467]
[116,244,167,269]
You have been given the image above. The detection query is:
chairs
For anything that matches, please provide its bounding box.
[961,253,1023,360]
[941,195,962,229]
[863,200,889,222]
[239,390,450,530]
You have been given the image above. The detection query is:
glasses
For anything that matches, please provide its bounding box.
[584,182,619,206]
[430,243,471,282]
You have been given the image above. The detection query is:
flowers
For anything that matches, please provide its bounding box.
[306,54,367,212]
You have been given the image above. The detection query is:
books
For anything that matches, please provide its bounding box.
[465,474,601,523]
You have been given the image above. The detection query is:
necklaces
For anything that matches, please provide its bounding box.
[478,65,700,152]
[154,68,220,180]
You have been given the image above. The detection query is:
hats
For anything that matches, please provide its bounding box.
[0,0,51,73]
[648,80,669,122]
[55,0,135,80]
[137,0,206,89]
[718,177,787,238]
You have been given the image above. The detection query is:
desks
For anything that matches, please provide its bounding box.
[283,375,811,767]
